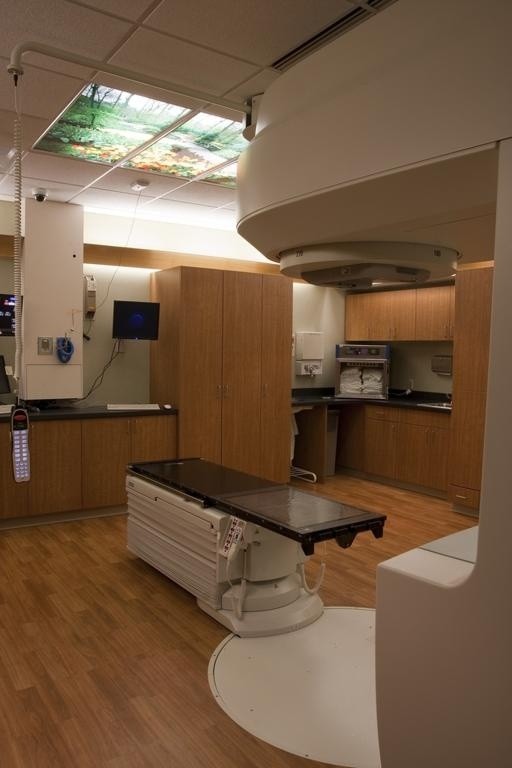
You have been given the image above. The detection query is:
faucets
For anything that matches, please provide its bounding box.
[446,394,452,400]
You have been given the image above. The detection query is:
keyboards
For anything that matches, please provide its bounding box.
[107,404,161,410]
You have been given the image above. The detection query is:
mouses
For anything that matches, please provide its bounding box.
[164,405,172,409]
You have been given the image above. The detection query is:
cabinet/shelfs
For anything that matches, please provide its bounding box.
[2,415,177,530]
[148,266,294,483]
[398,408,451,492]
[449,266,492,517]
[344,293,371,342]
[364,404,398,481]
[371,285,455,342]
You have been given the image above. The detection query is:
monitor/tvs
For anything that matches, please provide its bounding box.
[112,300,160,340]
[0,294,23,336]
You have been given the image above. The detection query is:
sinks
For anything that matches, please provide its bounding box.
[415,402,452,410]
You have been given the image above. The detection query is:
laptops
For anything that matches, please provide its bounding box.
[0,352,18,404]
